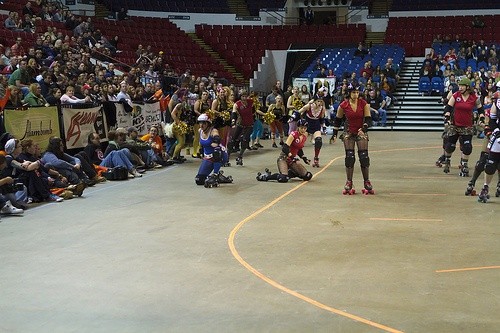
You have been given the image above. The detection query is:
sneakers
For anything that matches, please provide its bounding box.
[1,200,25,215]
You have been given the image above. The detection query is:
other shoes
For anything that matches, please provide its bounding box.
[309,115,500,149]
[37,151,202,203]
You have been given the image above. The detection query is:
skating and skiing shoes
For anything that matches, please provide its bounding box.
[343,180,356,195]
[477,185,490,203]
[219,130,290,169]
[495,181,500,197]
[435,154,445,167]
[443,156,451,173]
[312,157,319,168]
[204,170,234,188]
[464,179,476,196]
[361,180,375,195]
[459,158,469,177]
[256,168,271,181]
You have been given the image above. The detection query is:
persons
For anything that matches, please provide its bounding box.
[256,117,313,183]
[464,79,500,196]
[0,0,267,217]
[478,80,500,202]
[304,5,315,24]
[287,95,326,167]
[442,74,485,177]
[436,82,464,168]
[266,33,500,147]
[328,80,377,195]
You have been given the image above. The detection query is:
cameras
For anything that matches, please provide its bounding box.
[1,177,24,196]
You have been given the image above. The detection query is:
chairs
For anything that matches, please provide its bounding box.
[0,0,500,140]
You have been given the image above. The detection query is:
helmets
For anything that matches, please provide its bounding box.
[237,88,248,98]
[314,93,324,101]
[296,118,309,126]
[346,81,362,91]
[458,77,471,86]
[196,113,212,124]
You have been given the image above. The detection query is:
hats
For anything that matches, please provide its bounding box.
[128,126,138,135]
[5,139,15,154]
[115,128,128,136]
